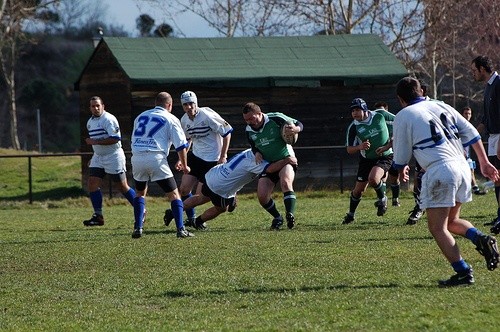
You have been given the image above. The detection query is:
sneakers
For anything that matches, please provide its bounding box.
[438,270,474,287]
[408,205,424,223]
[476,234,500,271]
[142,208,146,223]
[374,195,387,216]
[270,216,284,231]
[83,213,104,226]
[177,230,195,238]
[472,185,486,195]
[490,223,500,233]
[392,198,400,206]
[164,209,171,226]
[183,214,197,224]
[285,212,295,229]
[132,222,142,238]
[342,213,355,225]
[175,218,207,231]
[483,217,500,227]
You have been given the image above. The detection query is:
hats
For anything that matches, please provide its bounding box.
[181,91,197,105]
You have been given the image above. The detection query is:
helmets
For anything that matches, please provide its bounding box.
[350,98,368,111]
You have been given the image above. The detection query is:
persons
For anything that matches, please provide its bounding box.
[392,76,500,286]
[130,92,195,238]
[164,146,297,229]
[242,103,304,230]
[460,107,486,195]
[175,90,237,227]
[408,80,447,224]
[374,99,401,207]
[341,98,396,224]
[83,97,146,226]
[470,55,500,233]
